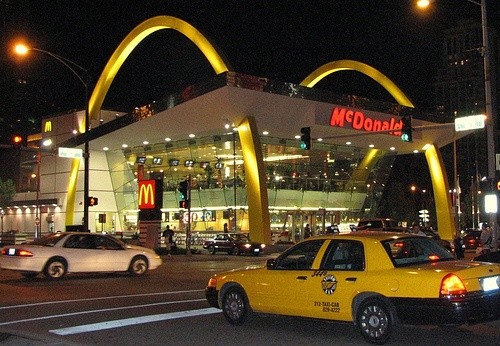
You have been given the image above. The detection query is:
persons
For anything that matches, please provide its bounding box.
[454,230,465,261]
[224,222,228,233]
[303,224,311,239]
[162,225,174,255]
[481,224,491,255]
[412,225,425,236]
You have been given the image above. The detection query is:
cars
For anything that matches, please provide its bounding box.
[0,231,163,280]
[203,232,266,256]
[462,231,482,250]
[206,231,500,344]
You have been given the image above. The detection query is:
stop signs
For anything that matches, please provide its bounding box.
[34,217,40,225]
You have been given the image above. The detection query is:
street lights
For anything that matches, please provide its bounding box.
[417,0,498,231]
[15,42,91,231]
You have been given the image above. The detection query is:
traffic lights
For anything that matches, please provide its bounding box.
[400,114,413,143]
[179,181,191,208]
[88,197,98,206]
[300,127,311,150]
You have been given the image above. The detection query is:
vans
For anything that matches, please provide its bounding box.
[357,218,404,233]
